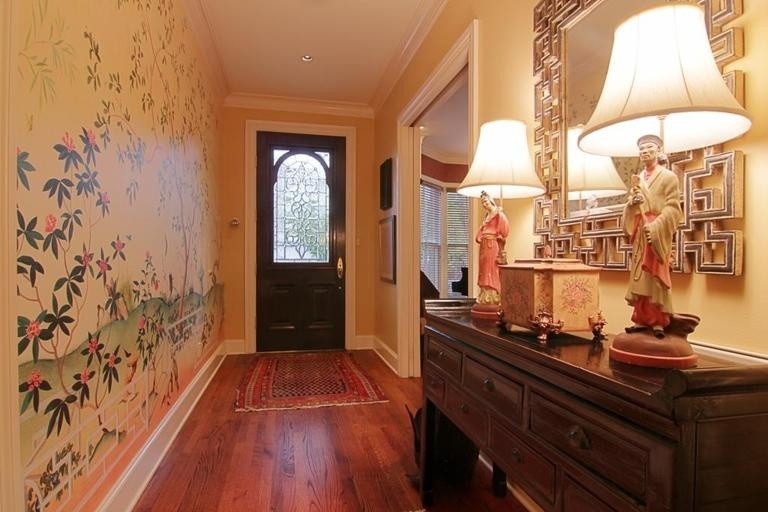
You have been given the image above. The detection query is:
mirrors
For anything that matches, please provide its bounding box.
[532,1,751,279]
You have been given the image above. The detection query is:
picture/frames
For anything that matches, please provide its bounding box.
[377,158,397,284]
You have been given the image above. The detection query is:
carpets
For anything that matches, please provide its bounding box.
[234,351,389,412]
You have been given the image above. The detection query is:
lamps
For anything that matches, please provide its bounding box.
[576,2,752,373]
[457,116,549,320]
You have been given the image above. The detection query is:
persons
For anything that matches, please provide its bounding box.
[474,192,510,304]
[620,133,684,339]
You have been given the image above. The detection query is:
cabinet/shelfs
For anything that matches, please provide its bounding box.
[419,299,767,511]
